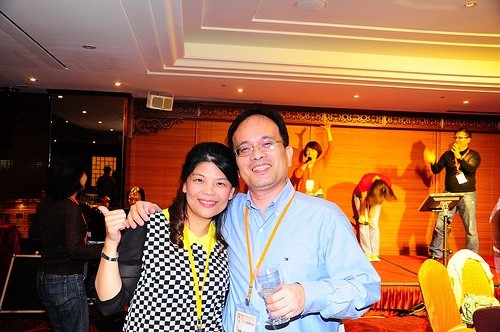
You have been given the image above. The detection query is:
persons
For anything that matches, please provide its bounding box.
[33,154,121,332]
[353,173,397,262]
[126,106,381,332]
[294,122,334,199]
[95,141,240,332]
[423,128,481,263]
[127,186,145,207]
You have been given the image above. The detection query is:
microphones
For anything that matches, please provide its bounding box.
[353,216,368,225]
[304,157,311,164]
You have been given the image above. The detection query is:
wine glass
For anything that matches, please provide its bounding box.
[254,266,290,326]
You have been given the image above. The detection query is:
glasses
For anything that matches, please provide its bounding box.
[234,138,287,158]
[453,137,466,139]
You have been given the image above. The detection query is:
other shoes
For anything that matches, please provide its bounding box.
[368,256,380,262]
[422,256,442,264]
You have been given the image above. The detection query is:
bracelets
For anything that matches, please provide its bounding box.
[101,247,119,261]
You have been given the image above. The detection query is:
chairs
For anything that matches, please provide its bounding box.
[472,307,500,332]
[447,249,500,329]
[417,259,476,332]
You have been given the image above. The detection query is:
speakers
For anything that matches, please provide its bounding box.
[146,96,173,112]
